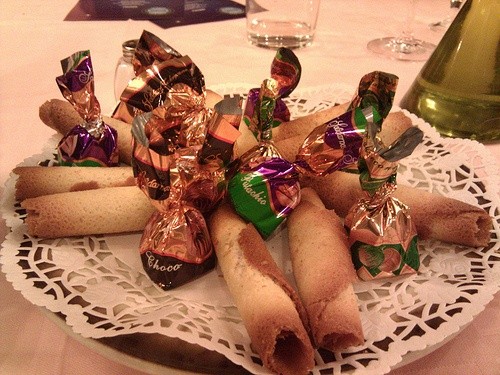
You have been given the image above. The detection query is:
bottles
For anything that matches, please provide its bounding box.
[398,0,500,144]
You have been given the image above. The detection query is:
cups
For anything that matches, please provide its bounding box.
[246,0,321,50]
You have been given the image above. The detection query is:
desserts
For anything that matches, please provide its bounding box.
[12,29,495,374]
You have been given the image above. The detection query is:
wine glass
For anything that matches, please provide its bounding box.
[367,0,437,62]
